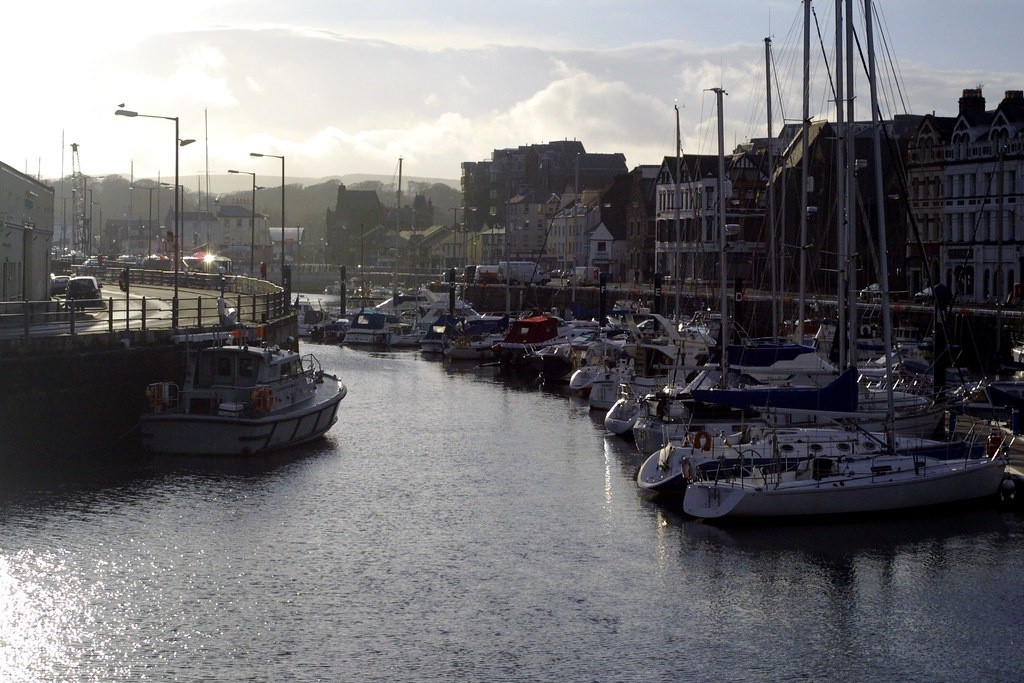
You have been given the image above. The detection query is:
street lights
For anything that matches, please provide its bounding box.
[161,182,185,271]
[128,185,173,271]
[71,187,93,256]
[227,170,266,278]
[113,109,198,326]
[250,152,286,287]
[71,176,88,261]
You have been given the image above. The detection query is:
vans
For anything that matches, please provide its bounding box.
[497,261,551,286]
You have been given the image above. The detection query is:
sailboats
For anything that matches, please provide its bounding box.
[297,1,1024,529]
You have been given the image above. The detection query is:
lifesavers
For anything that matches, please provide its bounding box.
[860,325,871,335]
[251,388,273,413]
[572,357,578,370]
[458,336,467,346]
[693,431,712,452]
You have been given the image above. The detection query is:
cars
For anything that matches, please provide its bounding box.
[913,287,933,304]
[64,275,103,309]
[859,283,882,301]
[49,274,71,298]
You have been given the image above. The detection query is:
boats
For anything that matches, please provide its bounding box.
[139,321,346,459]
